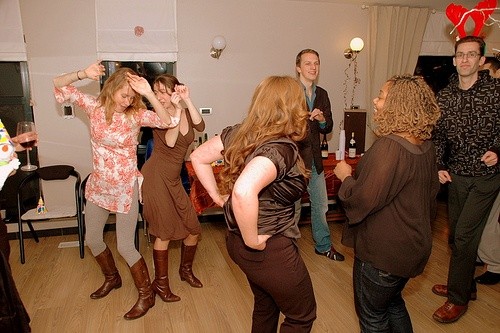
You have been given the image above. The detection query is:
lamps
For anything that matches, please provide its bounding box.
[343,37,364,59]
[209,36,225,60]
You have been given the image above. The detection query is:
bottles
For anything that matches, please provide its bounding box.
[198,137,202,146]
[335,149,341,160]
[348,132,356,159]
[203,133,207,143]
[191,140,197,153]
[320,134,328,159]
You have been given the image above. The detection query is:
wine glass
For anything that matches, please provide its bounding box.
[17,121,37,171]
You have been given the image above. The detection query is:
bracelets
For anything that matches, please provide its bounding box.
[77,70,81,80]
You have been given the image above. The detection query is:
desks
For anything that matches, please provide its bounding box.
[184,153,361,215]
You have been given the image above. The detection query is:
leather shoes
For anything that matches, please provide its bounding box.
[433,300,468,323]
[432,284,448,297]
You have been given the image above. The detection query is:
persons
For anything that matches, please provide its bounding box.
[139,74,206,302]
[291,49,346,261]
[189,75,317,333]
[53,60,180,319]
[334,74,440,333]
[429,36,500,324]
[0,119,32,333]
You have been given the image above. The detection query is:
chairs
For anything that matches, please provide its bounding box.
[16,164,84,264]
[80,174,140,253]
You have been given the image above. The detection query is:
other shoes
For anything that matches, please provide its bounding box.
[477,271,500,285]
[314,246,344,261]
[475,261,486,267]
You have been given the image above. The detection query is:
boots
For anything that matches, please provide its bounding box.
[124,254,156,319]
[152,248,181,302]
[179,241,203,289]
[90,244,122,299]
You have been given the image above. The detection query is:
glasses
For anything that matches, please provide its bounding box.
[456,51,480,59]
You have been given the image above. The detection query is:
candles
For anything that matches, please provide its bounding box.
[339,130,345,160]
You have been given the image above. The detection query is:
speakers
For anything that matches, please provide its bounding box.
[344,110,366,155]
